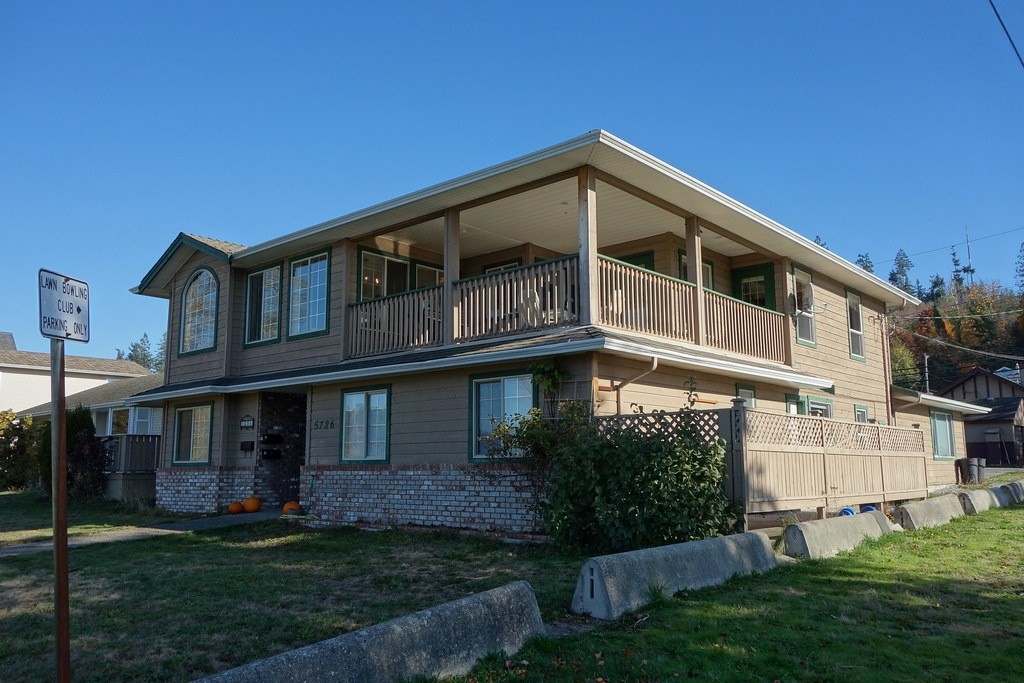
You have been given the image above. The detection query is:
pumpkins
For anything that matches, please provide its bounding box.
[283,501,300,514]
[243,496,260,512]
[227,502,242,514]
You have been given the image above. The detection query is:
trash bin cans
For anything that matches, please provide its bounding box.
[955,458,978,483]
[978,458,986,483]
